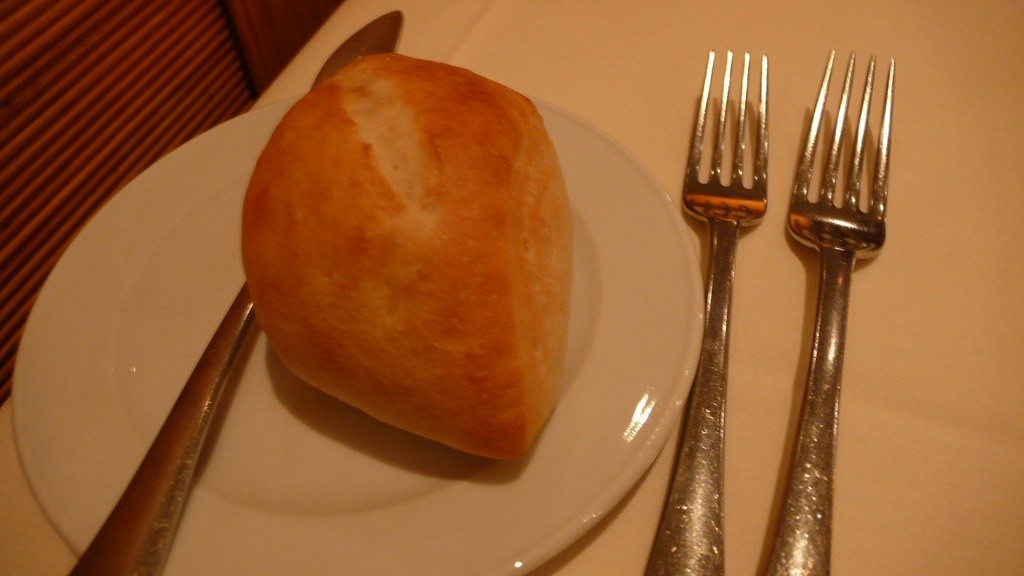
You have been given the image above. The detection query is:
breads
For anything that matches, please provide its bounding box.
[243,50,575,459]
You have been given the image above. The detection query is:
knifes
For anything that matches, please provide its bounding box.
[70,8,405,576]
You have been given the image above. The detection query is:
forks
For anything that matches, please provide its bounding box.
[643,50,772,576]
[755,45,896,576]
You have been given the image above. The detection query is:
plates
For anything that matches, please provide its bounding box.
[12,86,705,576]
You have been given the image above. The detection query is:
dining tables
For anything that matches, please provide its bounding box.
[0,0,1024,576]
